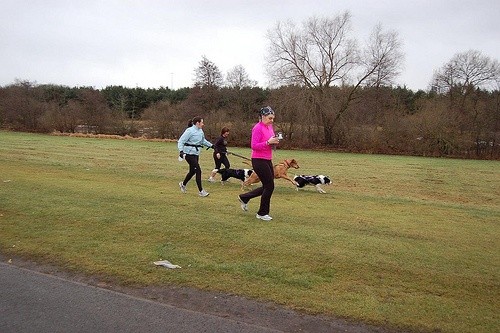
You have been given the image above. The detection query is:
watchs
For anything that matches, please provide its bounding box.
[267,140,269,145]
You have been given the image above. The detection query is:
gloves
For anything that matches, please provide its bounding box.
[211,145,215,148]
[180,151,186,158]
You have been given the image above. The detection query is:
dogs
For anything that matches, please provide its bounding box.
[211,168,254,187]
[293,175,332,194]
[240,159,299,191]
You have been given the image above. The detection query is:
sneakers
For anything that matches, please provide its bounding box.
[238,195,248,213]
[207,176,215,182]
[199,189,209,197]
[178,182,187,193]
[256,213,273,221]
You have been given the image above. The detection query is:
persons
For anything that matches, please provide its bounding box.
[238,107,280,220]
[208,127,230,184]
[178,117,214,197]
